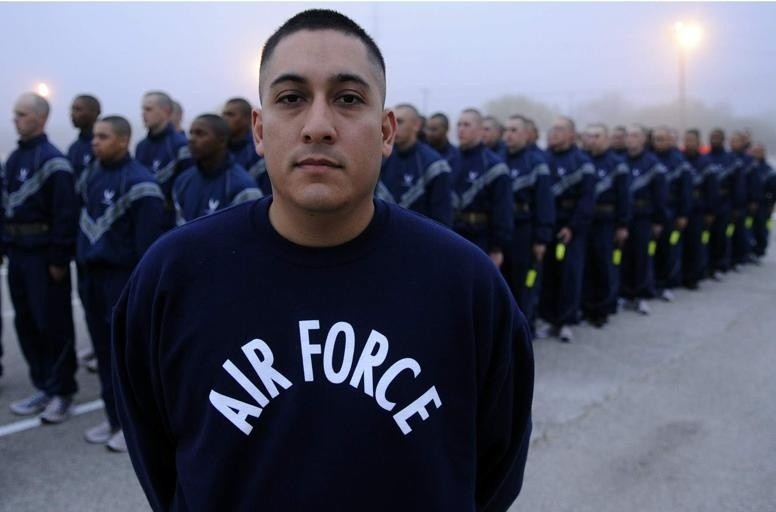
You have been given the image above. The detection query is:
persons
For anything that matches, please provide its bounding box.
[111,5,534,512]
[374,101,776,342]
[1,92,272,455]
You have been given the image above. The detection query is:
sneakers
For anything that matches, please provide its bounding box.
[10,391,70,423]
[85,423,127,452]
